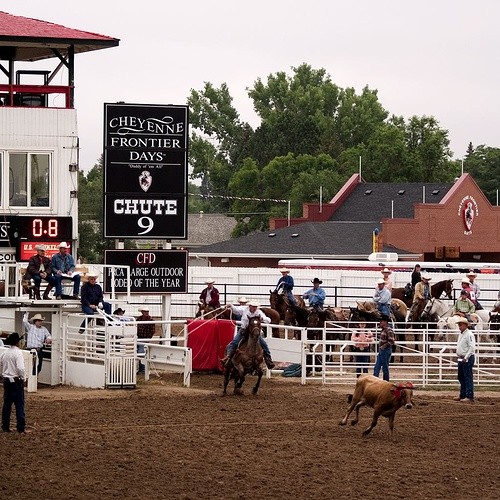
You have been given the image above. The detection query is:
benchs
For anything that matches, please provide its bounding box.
[16,262,87,300]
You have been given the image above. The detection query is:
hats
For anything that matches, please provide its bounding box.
[246,301,258,307]
[4,332,23,346]
[310,278,323,284]
[30,313,46,325]
[461,290,468,294]
[376,278,387,285]
[237,296,248,303]
[380,268,391,275]
[461,278,472,285]
[113,308,126,314]
[422,274,432,280]
[280,268,291,273]
[466,271,477,278]
[83,272,99,281]
[35,243,47,251]
[378,314,392,322]
[205,278,215,284]
[454,317,470,325]
[57,242,70,249]
[137,306,150,312]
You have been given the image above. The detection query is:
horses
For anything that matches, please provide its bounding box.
[221,314,267,396]
[197,290,500,364]
[389,279,455,306]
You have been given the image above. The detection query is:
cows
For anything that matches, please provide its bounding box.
[339,372,420,436]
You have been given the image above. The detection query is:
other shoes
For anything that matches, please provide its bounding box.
[79,330,84,334]
[453,395,471,402]
[56,294,62,299]
[73,294,81,299]
[43,296,52,299]
[36,295,41,300]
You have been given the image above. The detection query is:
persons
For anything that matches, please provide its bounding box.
[23,245,56,300]
[195,279,223,319]
[51,242,80,300]
[136,307,155,375]
[78,272,104,334]
[372,314,394,381]
[404,264,432,323]
[373,277,392,316]
[303,278,326,309]
[23,311,51,374]
[350,318,374,378]
[453,270,484,331]
[110,308,125,352]
[276,267,299,307]
[0,332,26,433]
[375,267,392,294]
[454,317,476,402]
[219,296,275,370]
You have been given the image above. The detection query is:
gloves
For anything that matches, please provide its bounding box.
[98,302,103,309]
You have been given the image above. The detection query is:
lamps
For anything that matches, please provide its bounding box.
[69,162,78,173]
[69,189,78,199]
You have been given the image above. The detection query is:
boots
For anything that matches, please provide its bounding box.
[220,353,233,363]
[264,355,275,369]
